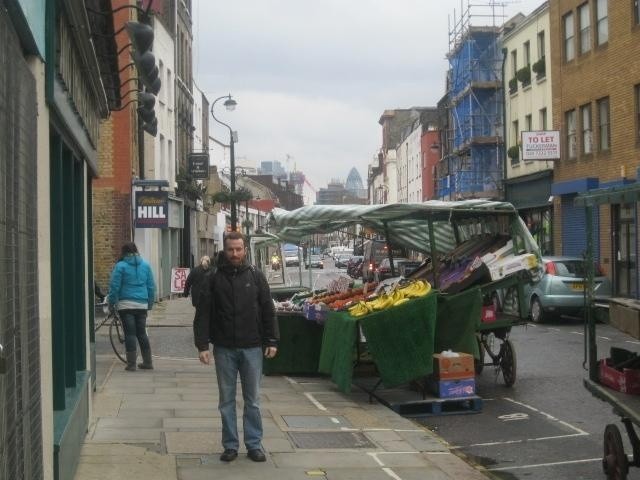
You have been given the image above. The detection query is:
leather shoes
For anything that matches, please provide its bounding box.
[247,450,265,461]
[221,449,236,461]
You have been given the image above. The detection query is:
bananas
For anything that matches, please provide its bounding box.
[348,279,431,316]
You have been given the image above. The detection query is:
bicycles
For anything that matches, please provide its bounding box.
[470,328,517,388]
[94,298,128,365]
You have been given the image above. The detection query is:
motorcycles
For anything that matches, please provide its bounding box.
[271,260,280,270]
[352,262,363,279]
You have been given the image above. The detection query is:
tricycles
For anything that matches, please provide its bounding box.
[569,186,639,479]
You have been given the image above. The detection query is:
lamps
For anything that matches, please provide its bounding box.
[83,4,162,138]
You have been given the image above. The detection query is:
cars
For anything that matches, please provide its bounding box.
[283,251,300,267]
[305,254,324,269]
[338,255,351,268]
[375,259,412,281]
[493,255,612,323]
[330,242,364,274]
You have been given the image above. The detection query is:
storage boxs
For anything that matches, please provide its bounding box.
[434,353,476,379]
[432,380,475,396]
[599,357,640,395]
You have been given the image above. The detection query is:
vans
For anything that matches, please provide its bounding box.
[363,240,408,281]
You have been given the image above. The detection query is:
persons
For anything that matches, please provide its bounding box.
[105,242,155,372]
[180,247,281,308]
[95,278,105,307]
[193,229,283,464]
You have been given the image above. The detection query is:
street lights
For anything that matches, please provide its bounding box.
[212,93,239,233]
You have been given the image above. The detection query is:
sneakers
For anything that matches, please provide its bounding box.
[138,363,152,368]
[125,365,136,371]
